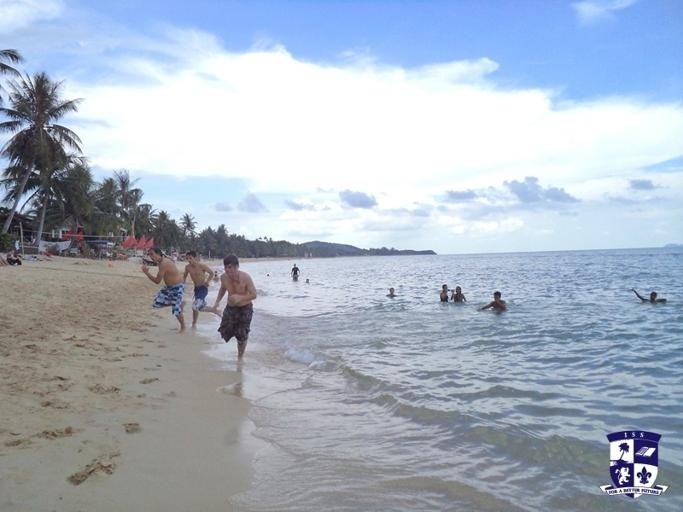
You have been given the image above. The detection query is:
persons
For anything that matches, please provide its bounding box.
[475,291,506,314]
[140,247,185,333]
[386,288,396,296]
[439,285,449,302]
[631,288,666,303]
[182,250,222,331]
[6,248,22,267]
[210,254,257,360]
[290,264,300,282]
[449,286,466,303]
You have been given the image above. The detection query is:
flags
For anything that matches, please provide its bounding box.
[121,235,153,250]
